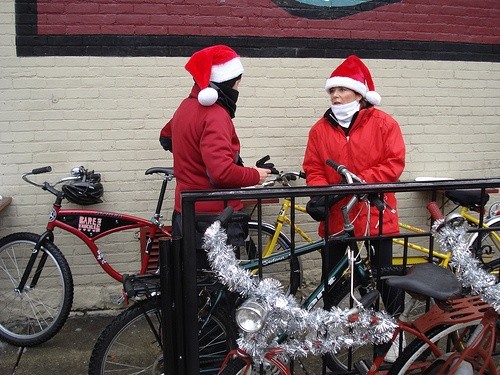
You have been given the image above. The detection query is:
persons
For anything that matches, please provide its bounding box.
[159,45,271,375]
[303,55,407,361]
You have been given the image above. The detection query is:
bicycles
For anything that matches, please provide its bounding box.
[0,153,500,375]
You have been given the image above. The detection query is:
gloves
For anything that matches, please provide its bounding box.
[256,155,278,174]
[306,197,327,221]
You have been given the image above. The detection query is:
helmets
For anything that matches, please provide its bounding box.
[62,182,103,204]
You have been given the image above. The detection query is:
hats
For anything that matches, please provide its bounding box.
[325,55,381,105]
[185,45,243,107]
